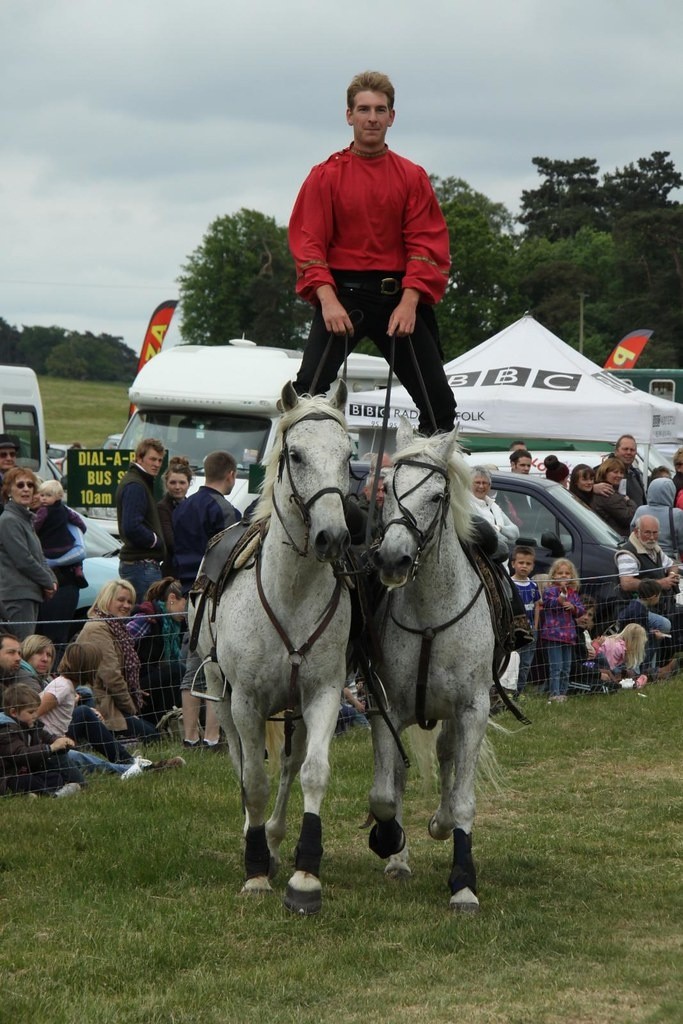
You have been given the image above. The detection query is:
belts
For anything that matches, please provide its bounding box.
[331,277,406,298]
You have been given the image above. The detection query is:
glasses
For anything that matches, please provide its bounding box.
[0,452,19,458]
[640,530,659,537]
[14,482,34,489]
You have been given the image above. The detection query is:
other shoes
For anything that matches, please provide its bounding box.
[76,568,88,588]
[54,783,81,800]
[657,657,680,681]
[203,737,228,751]
[183,738,204,746]
[507,694,526,703]
[154,757,184,769]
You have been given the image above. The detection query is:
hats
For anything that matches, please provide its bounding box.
[0,434,20,452]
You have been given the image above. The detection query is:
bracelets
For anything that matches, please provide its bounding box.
[669,572,676,574]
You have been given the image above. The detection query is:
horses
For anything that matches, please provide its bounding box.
[186,376,536,919]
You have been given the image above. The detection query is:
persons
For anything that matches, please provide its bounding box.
[0,425,683,798]
[281,67,459,442]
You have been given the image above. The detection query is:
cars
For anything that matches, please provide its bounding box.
[43,433,127,648]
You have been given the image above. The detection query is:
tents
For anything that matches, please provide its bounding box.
[343,310,682,506]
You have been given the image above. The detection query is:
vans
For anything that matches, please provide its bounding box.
[81,329,405,546]
[347,459,630,655]
[0,365,63,488]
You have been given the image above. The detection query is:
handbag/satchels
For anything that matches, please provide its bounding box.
[496,651,520,691]
